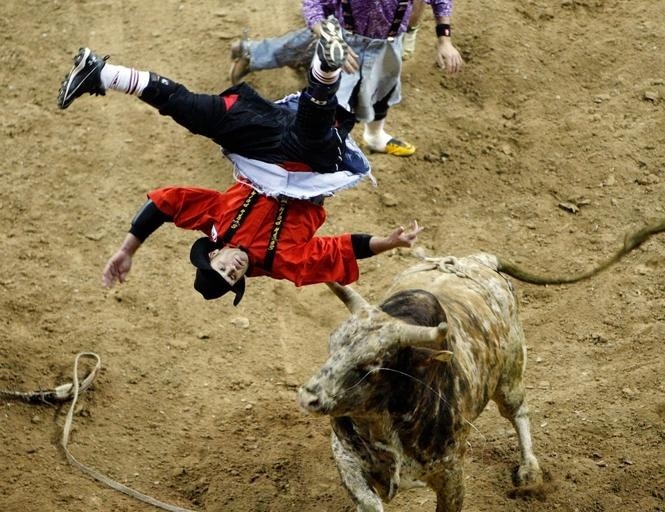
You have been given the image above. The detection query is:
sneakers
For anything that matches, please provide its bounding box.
[58,47,110,109]
[360,134,415,157]
[229,42,250,86]
[317,15,348,72]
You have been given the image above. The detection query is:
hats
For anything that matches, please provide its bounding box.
[190,238,245,306]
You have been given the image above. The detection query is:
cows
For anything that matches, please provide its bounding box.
[296,217,664,512]
